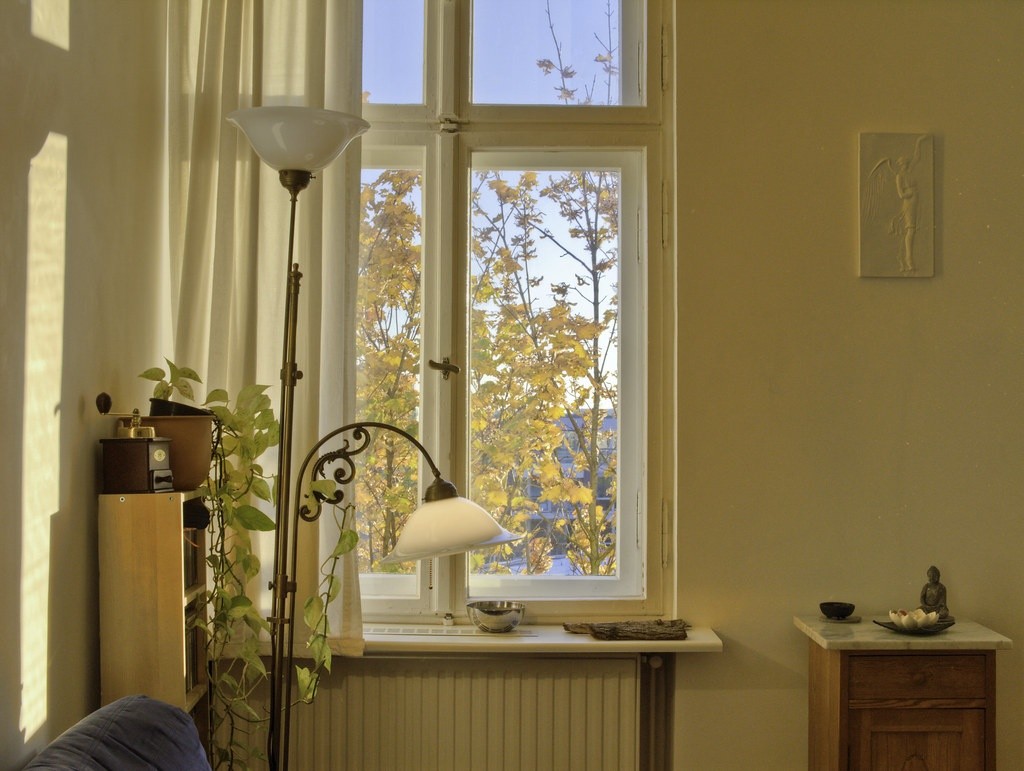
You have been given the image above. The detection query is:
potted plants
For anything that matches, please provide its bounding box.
[137,363,353,724]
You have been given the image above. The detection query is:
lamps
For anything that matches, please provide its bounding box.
[221,107,523,771]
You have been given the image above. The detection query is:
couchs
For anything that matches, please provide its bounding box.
[21,694,213,771]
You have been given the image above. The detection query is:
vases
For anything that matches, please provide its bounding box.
[121,414,214,486]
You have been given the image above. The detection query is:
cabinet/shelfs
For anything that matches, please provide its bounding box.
[92,489,208,728]
[793,615,1013,771]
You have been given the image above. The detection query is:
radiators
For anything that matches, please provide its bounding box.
[247,657,643,771]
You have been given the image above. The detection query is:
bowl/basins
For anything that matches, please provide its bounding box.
[820,602,855,619]
[467,601,526,633]
